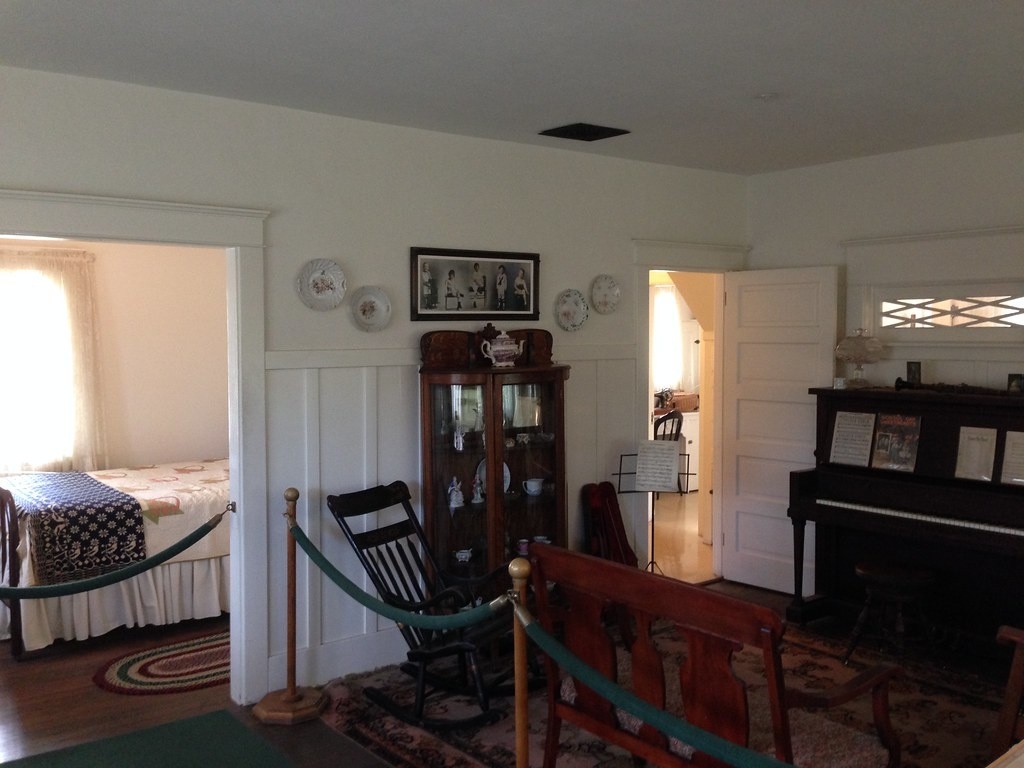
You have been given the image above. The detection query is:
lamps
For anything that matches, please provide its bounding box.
[835,328,882,389]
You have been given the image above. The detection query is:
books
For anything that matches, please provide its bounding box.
[1001,431,1024,485]
[635,440,679,493]
[872,415,921,471]
[830,412,876,466]
[956,427,997,480]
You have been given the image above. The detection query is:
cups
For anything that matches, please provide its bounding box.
[834,377,846,389]
[519,539,528,555]
[522,479,544,496]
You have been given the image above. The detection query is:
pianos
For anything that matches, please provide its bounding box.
[785,384,1024,661]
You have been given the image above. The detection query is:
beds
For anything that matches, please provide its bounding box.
[0,458,230,660]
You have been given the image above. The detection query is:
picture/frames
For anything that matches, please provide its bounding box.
[410,247,540,321]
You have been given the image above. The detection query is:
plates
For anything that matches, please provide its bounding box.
[476,458,511,494]
[350,286,391,332]
[297,259,347,311]
[554,289,589,331]
[591,274,622,315]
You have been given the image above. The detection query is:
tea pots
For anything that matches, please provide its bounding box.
[480,331,526,367]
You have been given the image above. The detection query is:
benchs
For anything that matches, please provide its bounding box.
[526,541,1024,768]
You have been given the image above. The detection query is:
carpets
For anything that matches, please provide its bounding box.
[0,709,296,768]
[92,627,230,696]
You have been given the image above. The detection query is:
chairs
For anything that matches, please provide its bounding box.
[328,481,538,726]
[653,409,683,500]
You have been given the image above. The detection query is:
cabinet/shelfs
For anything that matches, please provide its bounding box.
[418,322,569,583]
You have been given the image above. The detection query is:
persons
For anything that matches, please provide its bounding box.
[514,268,528,306]
[421,262,433,309]
[471,263,483,307]
[445,270,462,310]
[497,265,508,310]
